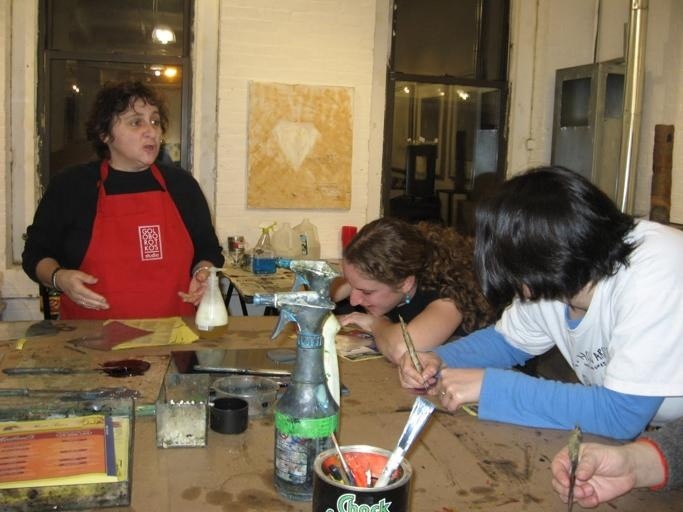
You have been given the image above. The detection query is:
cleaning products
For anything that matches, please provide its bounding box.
[194,266,228,339]
[251,221,275,275]
[253,290,340,502]
[274,256,342,407]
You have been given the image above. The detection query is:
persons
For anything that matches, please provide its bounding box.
[328,212,538,380]
[396,161,683,442]
[549,413,683,510]
[22,77,227,327]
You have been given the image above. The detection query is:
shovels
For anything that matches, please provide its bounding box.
[2,359,150,377]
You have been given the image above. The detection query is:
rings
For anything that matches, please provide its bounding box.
[82,301,90,309]
[439,392,446,397]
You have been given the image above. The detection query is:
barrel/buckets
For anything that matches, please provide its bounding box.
[272,222,303,259]
[293,218,322,261]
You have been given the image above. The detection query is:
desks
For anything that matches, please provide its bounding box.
[219,257,342,316]
[0,319,649,511]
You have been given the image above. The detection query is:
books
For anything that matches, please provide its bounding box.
[0,412,128,490]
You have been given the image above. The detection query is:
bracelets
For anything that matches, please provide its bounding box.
[193,266,212,276]
[51,265,66,299]
